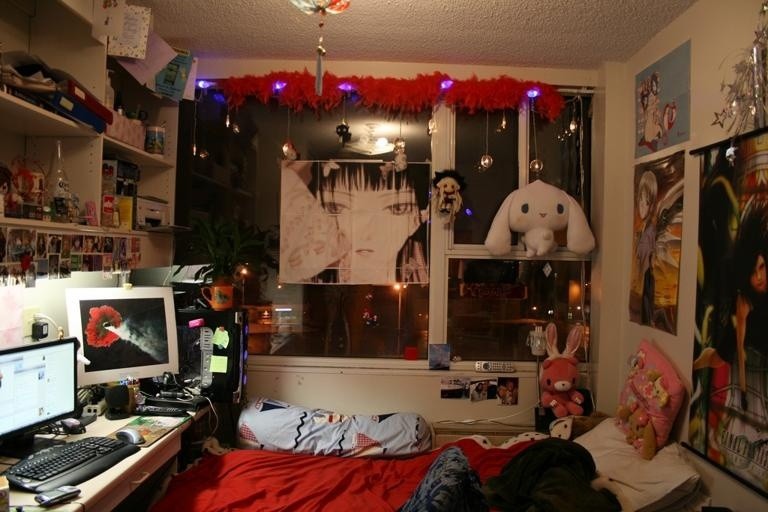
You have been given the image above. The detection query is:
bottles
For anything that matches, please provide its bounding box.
[46,140,70,224]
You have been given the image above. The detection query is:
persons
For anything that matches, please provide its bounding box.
[634,171,680,332]
[280,159,428,283]
[428,169,465,223]
[638,72,666,143]
[448,379,518,406]
[0,234,100,278]
[697,202,767,412]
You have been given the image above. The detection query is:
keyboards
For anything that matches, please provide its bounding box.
[7,437,141,494]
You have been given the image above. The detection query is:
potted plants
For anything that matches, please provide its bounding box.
[177,218,282,312]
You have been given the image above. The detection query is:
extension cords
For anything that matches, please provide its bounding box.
[83,395,107,416]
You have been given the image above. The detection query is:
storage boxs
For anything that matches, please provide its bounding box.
[106,106,148,151]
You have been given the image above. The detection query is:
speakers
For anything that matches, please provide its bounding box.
[105,385,130,420]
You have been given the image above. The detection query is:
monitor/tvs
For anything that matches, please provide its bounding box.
[0,336,78,458]
[65,287,179,389]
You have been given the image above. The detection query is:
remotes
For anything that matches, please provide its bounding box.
[134,406,186,417]
[475,361,515,373]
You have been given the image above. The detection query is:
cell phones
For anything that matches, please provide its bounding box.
[34,486,81,507]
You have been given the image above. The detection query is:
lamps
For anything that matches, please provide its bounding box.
[289,0,351,96]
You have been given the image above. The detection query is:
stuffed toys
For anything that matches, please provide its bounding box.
[539,321,588,417]
[484,178,596,259]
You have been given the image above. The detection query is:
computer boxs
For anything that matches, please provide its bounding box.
[139,308,248,404]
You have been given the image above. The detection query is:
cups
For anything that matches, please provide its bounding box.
[201,286,233,311]
[143,124,165,158]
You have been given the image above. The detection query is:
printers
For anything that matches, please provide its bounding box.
[136,196,192,234]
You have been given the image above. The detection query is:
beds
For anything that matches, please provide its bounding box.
[144,397,711,512]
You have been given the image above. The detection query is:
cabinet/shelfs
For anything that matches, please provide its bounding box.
[0,0,171,238]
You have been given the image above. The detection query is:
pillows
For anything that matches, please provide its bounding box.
[236,396,432,462]
[611,338,688,460]
[572,412,700,512]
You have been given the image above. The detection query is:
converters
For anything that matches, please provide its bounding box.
[32,323,49,339]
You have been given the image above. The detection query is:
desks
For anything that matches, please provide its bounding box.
[0,401,220,512]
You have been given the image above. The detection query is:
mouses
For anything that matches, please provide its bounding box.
[62,417,86,434]
[116,430,145,445]
